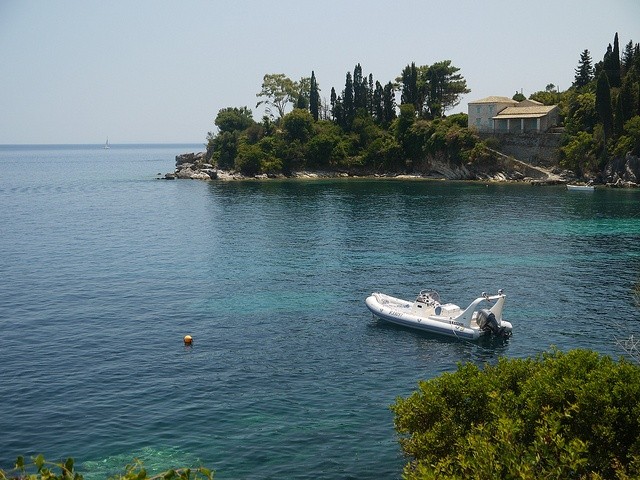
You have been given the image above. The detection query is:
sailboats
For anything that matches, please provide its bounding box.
[104,137,110,149]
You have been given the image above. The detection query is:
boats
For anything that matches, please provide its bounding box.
[566,184,598,191]
[365,288,513,344]
